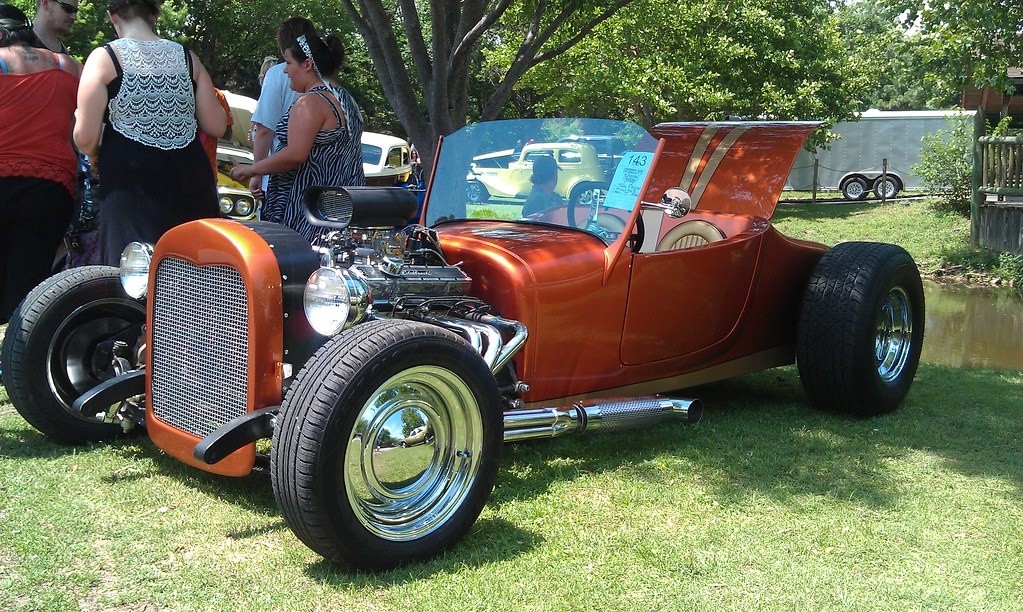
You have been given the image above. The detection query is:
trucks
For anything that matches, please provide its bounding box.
[728,107,979,201]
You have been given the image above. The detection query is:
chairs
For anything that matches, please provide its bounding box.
[533,203,754,252]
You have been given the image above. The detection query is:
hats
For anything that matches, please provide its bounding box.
[530,139,534,142]
[533,155,563,171]
[517,140,521,143]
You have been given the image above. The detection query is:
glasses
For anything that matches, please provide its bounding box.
[53,0,79,14]
[412,153,417,155]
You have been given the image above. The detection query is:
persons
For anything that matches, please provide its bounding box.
[30,0,79,56]
[248,56,280,145]
[513,140,523,160]
[197,86,233,191]
[248,17,316,221]
[227,30,366,246]
[522,156,568,218]
[0,0,83,325]
[410,150,420,164]
[73,0,226,265]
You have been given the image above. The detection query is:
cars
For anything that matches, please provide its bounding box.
[72,89,410,221]
[0,117,926,573]
[464,136,634,206]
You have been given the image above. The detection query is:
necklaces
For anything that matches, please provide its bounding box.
[34,29,60,48]
